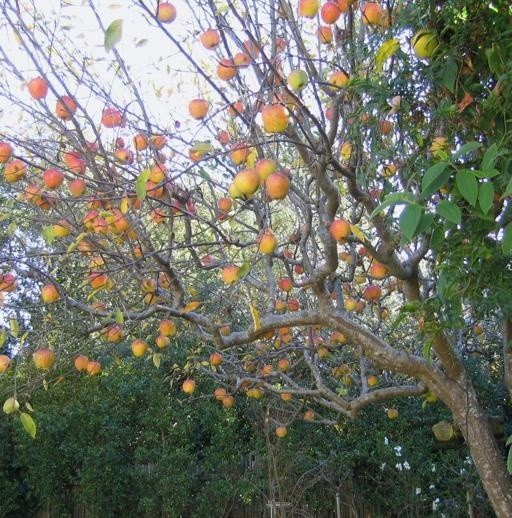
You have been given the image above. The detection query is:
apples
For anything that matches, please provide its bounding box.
[0,0,502,443]
